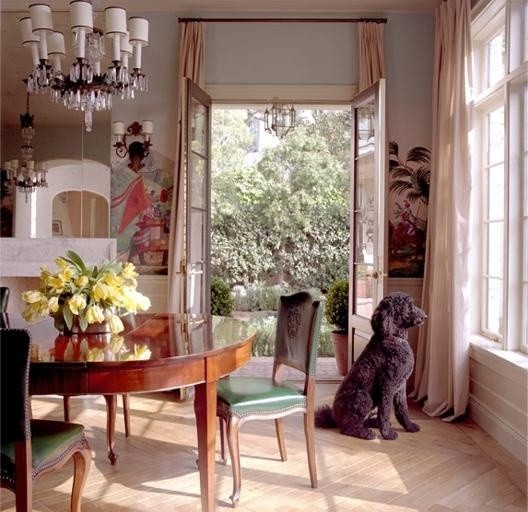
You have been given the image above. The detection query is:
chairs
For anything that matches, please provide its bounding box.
[195,290,324,508]
[1,328,91,511]
[0,285,11,328]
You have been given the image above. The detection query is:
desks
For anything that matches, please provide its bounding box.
[1,310,257,511]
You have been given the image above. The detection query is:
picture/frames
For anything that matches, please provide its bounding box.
[52,219,64,236]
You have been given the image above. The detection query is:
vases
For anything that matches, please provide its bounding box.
[51,315,108,333]
[70,332,112,348]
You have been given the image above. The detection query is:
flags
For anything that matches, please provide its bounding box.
[111,175,150,234]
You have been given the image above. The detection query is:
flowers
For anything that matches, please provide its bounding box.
[44,336,153,362]
[20,248,151,333]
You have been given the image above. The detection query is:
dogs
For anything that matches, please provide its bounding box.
[313,291,429,442]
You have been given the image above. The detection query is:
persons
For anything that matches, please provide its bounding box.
[397,201,417,233]
[130,205,171,233]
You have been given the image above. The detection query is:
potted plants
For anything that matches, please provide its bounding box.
[323,280,347,375]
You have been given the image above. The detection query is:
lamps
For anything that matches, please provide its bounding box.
[18,0,150,132]
[110,119,155,172]
[262,104,296,139]
[0,79,51,194]
